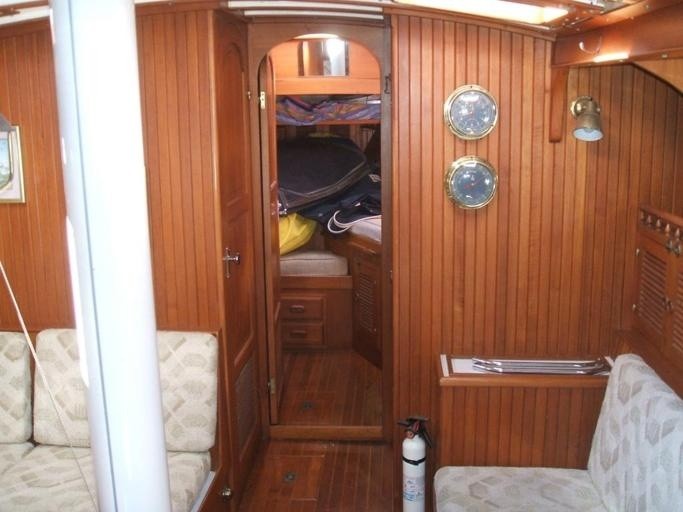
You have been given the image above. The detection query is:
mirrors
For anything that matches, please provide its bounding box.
[297,39,350,77]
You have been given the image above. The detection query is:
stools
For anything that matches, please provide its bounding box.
[276,250,353,351]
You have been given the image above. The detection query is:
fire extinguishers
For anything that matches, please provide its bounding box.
[396,415,433,512]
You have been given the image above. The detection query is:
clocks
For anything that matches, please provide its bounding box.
[445,156,498,210]
[443,84,498,141]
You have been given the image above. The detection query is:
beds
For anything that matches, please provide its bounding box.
[326,215,384,368]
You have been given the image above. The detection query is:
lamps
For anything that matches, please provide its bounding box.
[570,96,604,142]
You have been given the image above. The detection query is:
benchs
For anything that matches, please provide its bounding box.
[435,352,680,510]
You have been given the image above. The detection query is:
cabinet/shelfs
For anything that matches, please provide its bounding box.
[625,212,683,377]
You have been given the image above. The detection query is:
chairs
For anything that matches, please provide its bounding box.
[0,329,220,512]
[0,332,30,473]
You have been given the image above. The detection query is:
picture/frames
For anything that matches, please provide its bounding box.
[0,125,26,204]
